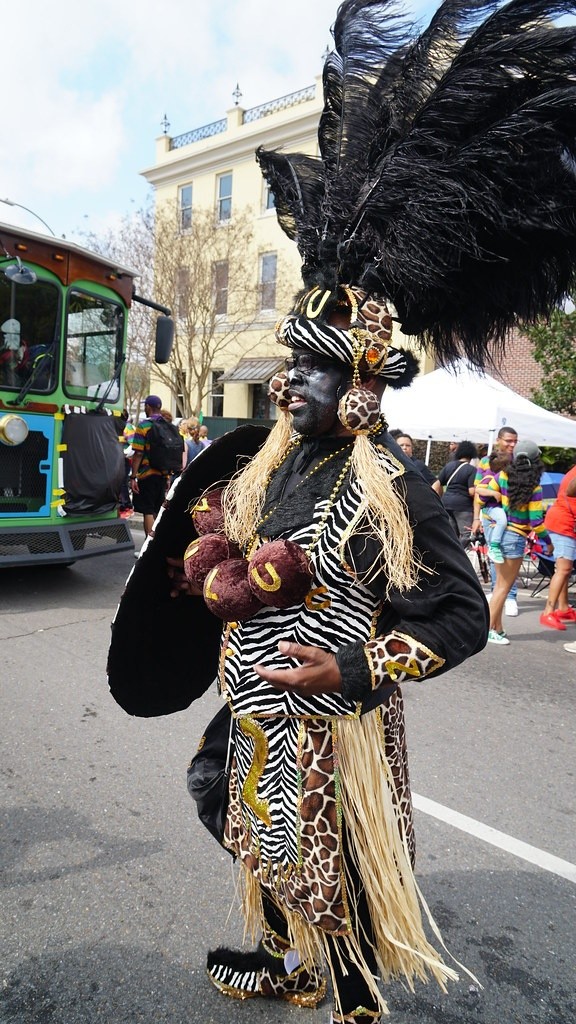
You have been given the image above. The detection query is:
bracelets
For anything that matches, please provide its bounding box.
[130,476,136,479]
[474,518,480,520]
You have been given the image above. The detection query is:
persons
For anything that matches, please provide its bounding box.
[390,429,443,498]
[476,441,551,644]
[107,283,491,1024]
[472,427,518,616]
[0,306,81,391]
[540,466,576,631]
[118,409,136,519]
[438,442,488,548]
[160,410,213,494]
[475,453,513,564]
[131,395,170,558]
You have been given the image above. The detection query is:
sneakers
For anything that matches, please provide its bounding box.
[487,546,505,564]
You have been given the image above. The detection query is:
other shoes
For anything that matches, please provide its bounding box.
[556,607,576,621]
[540,611,567,630]
[487,593,495,602]
[134,552,140,558]
[205,915,327,1009]
[488,629,510,645]
[563,641,576,653]
[330,1006,382,1024]
[504,598,519,616]
[121,508,134,519]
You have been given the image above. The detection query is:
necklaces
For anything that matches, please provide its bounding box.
[241,422,389,560]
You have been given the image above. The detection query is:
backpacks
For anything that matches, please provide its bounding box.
[24,345,55,384]
[0,338,30,374]
[145,416,183,475]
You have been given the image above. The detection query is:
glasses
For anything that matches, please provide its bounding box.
[285,354,336,372]
[500,437,519,444]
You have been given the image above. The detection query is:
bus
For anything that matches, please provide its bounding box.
[0,218,175,575]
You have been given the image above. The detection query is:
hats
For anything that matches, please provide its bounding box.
[140,395,162,409]
[513,440,543,466]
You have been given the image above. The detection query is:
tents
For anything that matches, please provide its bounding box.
[381,357,576,468]
